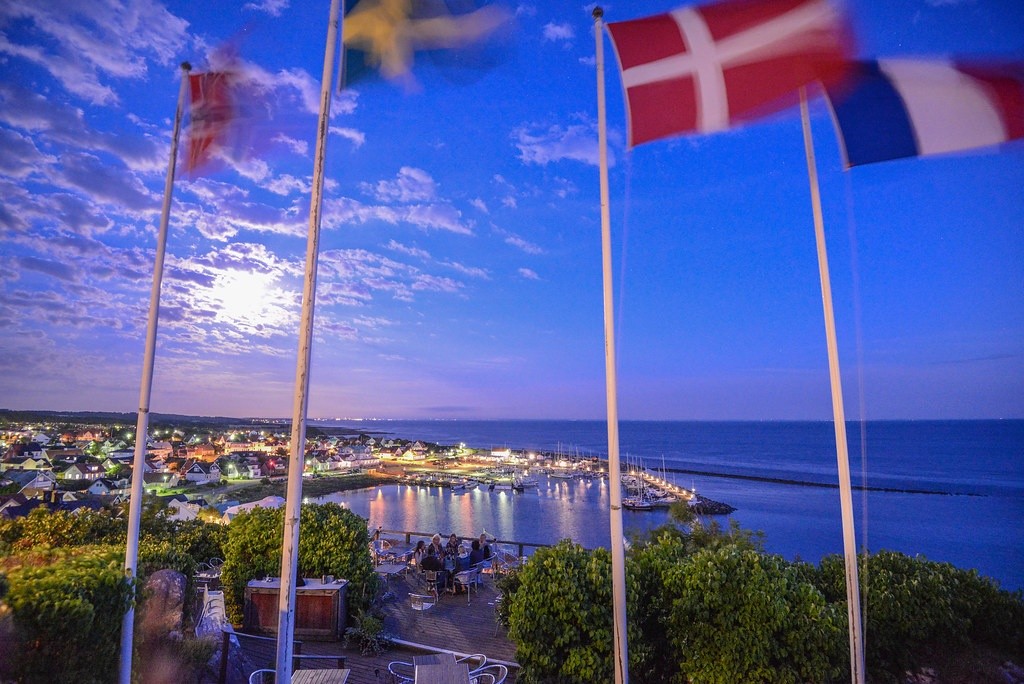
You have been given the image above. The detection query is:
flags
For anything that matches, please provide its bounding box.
[186,71,230,170]
[603,1,858,152]
[820,56,1024,171]
[337,0,517,97]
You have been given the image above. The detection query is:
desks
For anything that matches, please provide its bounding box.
[374,562,408,593]
[413,653,458,665]
[291,669,351,684]
[438,547,475,581]
[384,538,418,566]
[415,664,469,684]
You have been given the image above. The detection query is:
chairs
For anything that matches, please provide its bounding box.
[388,662,414,684]
[469,665,508,684]
[363,529,528,640]
[249,669,276,684]
[456,654,487,671]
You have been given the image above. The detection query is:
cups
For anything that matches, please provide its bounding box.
[322,575,326,584]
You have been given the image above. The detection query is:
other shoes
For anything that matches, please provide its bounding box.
[444,588,452,592]
[428,588,436,595]
[460,589,467,594]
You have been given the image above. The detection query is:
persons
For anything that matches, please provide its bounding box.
[445,533,459,556]
[420,544,470,596]
[374,527,381,540]
[428,534,443,556]
[414,540,428,564]
[469,534,501,571]
